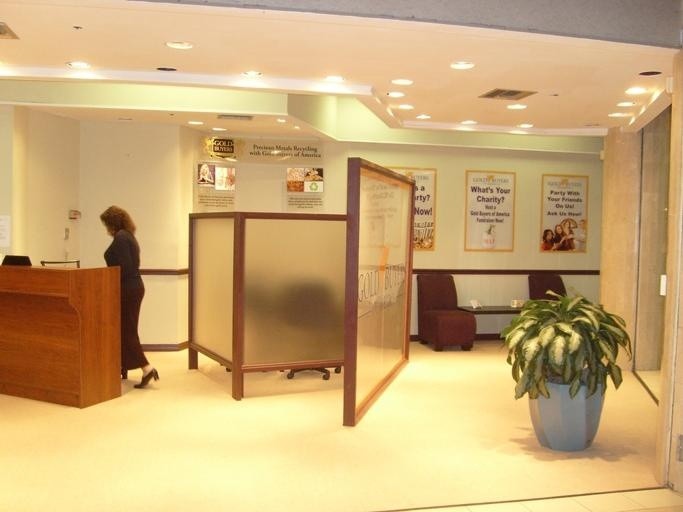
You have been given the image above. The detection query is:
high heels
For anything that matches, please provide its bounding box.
[121,367,128,379]
[133,368,160,389]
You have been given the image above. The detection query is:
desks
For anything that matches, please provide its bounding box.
[459,304,524,335]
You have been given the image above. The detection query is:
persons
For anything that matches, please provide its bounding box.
[574,219,585,250]
[560,221,576,251]
[197,164,213,184]
[98,205,158,389]
[551,224,571,251]
[539,229,560,251]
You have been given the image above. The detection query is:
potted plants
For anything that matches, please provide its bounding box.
[496,286,633,450]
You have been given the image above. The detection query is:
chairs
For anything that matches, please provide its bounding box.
[416,274,476,351]
[527,273,567,299]
